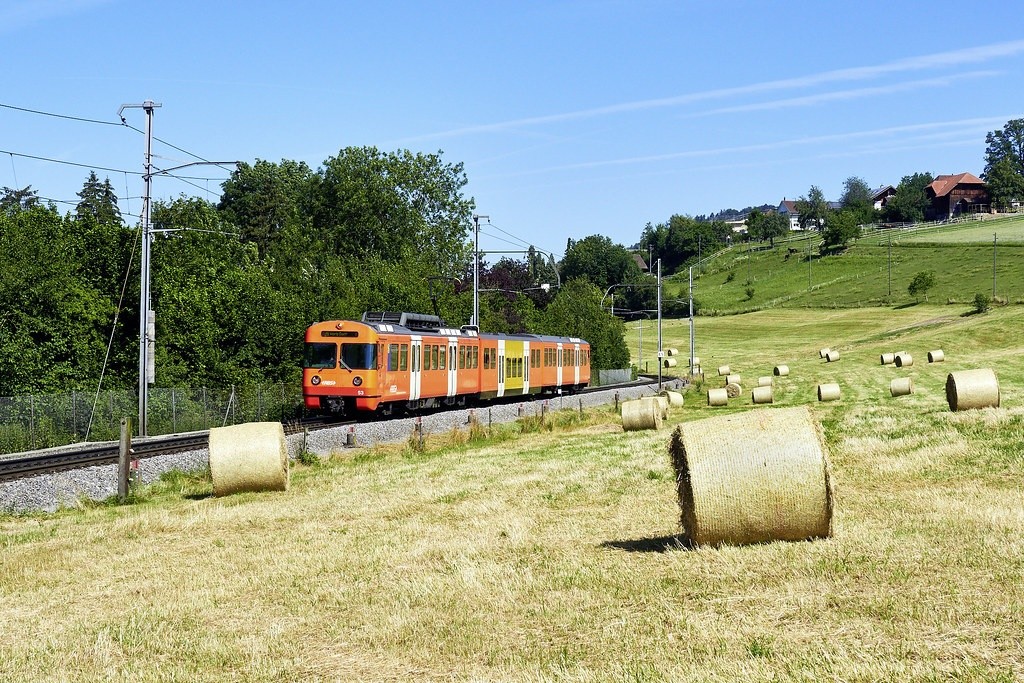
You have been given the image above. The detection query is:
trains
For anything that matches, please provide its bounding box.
[302,310,591,424]
